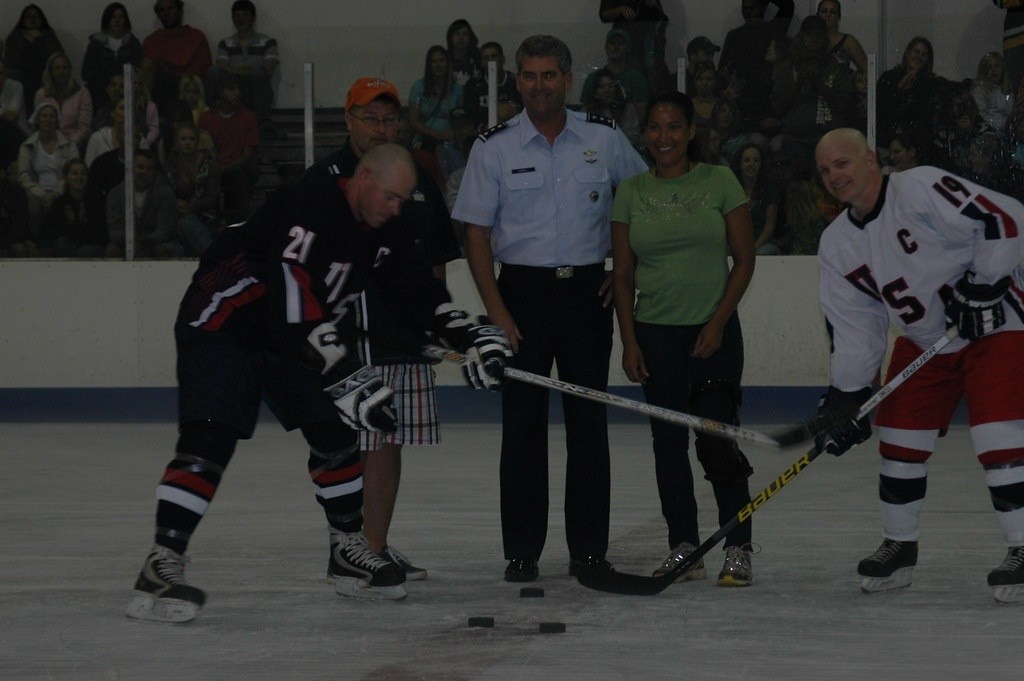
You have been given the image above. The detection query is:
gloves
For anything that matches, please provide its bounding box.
[816,386,874,456]
[322,361,399,433]
[944,270,1013,342]
[461,323,512,394]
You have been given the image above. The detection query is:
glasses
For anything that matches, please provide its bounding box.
[348,111,401,125]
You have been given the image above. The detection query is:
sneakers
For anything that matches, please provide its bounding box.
[569,554,617,582]
[716,542,762,585]
[858,538,919,590]
[653,541,707,583]
[326,523,407,598]
[379,545,427,579]
[125,543,204,622]
[987,546,1024,602]
[504,555,539,580]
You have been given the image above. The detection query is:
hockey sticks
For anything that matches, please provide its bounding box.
[573,324,963,599]
[418,342,839,449]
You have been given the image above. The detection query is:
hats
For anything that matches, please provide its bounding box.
[607,28,631,43]
[346,78,402,112]
[687,36,720,52]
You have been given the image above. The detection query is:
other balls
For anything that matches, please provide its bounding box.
[520,588,544,597]
[539,621,566,633]
[468,616,495,627]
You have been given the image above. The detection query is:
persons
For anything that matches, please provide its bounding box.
[124,144,514,622]
[0,0,281,257]
[812,128,1024,607]
[270,77,458,580]
[580,0,1024,255]
[610,91,762,587]
[407,18,524,213]
[449,34,649,583]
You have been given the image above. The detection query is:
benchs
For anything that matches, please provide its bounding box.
[250,104,348,179]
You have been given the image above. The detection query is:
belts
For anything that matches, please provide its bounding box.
[499,262,606,279]
